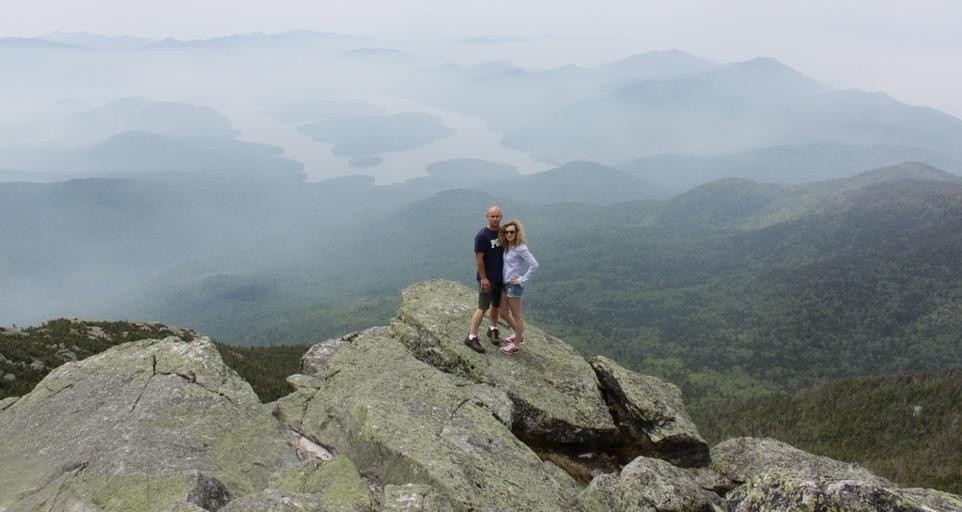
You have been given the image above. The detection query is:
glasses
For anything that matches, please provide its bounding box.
[504,230,515,234]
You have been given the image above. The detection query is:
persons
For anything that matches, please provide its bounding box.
[464,207,504,353]
[498,219,539,355]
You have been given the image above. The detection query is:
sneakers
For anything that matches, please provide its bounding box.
[503,336,525,344]
[465,333,485,353]
[501,344,520,354]
[487,327,500,344]
